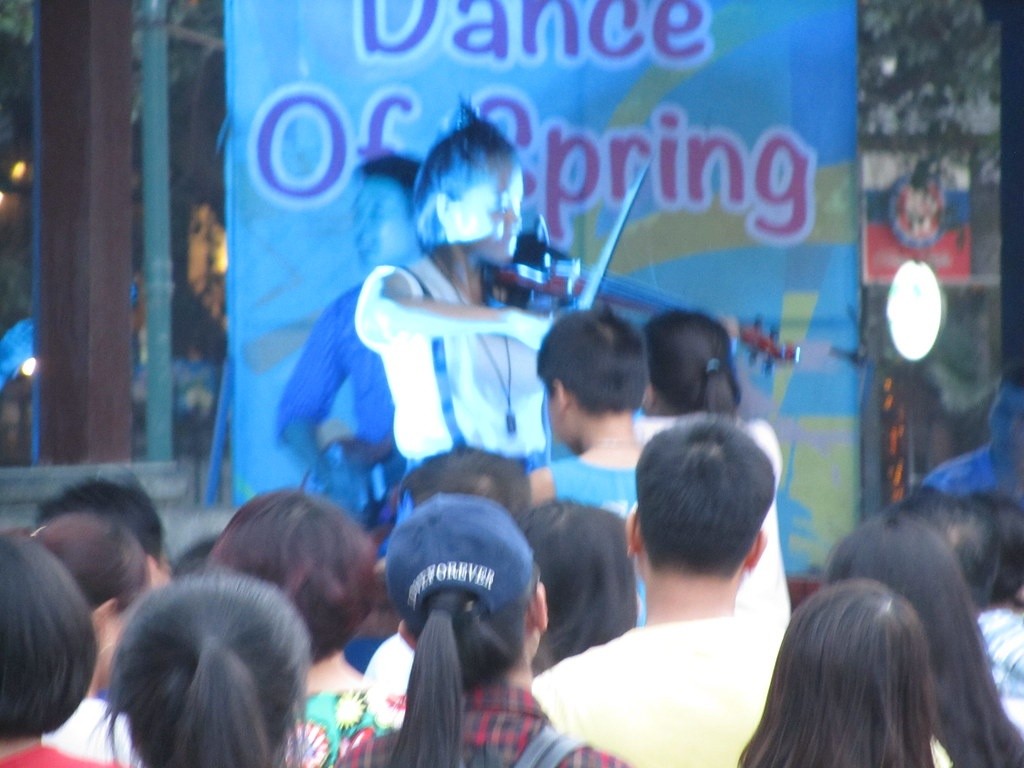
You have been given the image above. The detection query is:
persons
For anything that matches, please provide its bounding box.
[528,419,792,768]
[919,372,1022,497]
[820,516,1023,766]
[735,575,937,768]
[404,449,530,519]
[634,309,792,610]
[333,493,635,768]
[96,570,308,768]
[979,489,1022,730]
[882,491,999,617]
[32,477,170,589]
[526,306,650,623]
[206,489,401,768]
[275,96,578,478]
[0,534,122,768]
[6,513,151,768]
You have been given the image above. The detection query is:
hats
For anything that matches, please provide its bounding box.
[386,490,536,637]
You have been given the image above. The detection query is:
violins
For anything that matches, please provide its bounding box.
[479,232,802,378]
